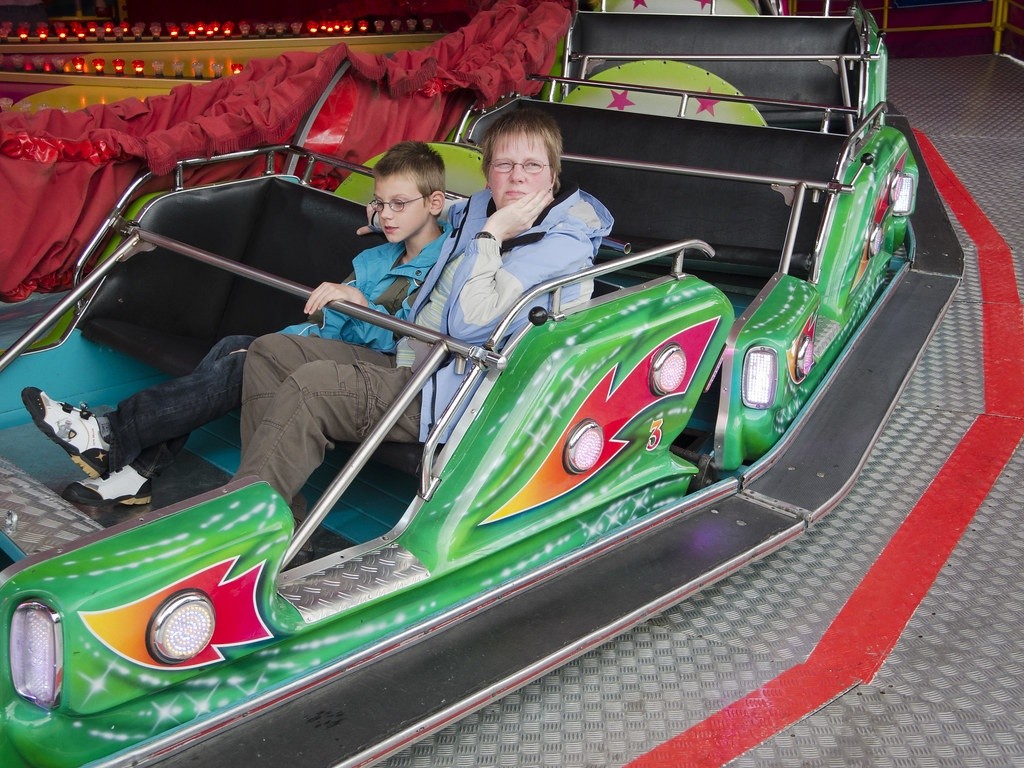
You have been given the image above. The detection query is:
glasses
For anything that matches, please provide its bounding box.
[368,193,430,212]
[489,158,552,174]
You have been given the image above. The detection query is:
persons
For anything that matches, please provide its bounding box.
[22,142,445,505]
[228,110,614,572]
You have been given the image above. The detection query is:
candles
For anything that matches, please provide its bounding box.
[0,16,433,80]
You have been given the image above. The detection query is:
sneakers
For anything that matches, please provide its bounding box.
[62,462,151,505]
[21,386,111,481]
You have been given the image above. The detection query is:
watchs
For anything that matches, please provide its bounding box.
[475,232,495,240]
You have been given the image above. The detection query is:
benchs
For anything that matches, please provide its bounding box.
[562,10,866,125]
[462,95,851,273]
[75,176,624,478]
